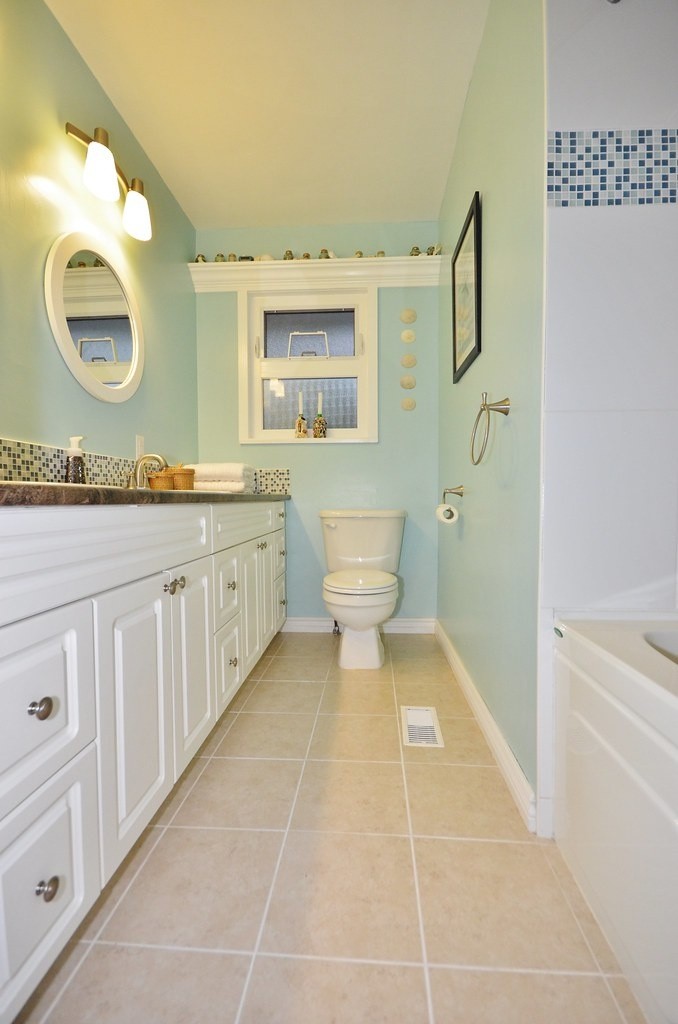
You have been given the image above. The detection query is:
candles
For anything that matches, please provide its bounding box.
[318,392,322,414]
[299,391,303,414]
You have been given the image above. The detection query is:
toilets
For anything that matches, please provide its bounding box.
[317,510,409,670]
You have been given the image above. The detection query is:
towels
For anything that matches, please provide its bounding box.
[183,463,256,492]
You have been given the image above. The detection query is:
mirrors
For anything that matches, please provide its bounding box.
[44,230,146,405]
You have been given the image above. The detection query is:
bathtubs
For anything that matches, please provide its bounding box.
[552,620,678,1024]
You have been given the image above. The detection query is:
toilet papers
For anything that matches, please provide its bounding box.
[435,504,459,524]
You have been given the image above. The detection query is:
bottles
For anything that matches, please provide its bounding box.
[215,253,224,262]
[283,251,293,260]
[319,249,330,258]
[303,253,310,259]
[228,254,237,262]
[409,247,421,256]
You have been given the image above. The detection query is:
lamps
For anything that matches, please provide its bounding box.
[66,120,153,242]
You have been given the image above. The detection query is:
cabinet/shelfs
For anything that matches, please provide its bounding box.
[271,500,288,636]
[213,543,243,721]
[241,532,274,684]
[91,555,217,890]
[2,595,102,1024]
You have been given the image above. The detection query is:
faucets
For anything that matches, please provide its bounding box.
[134,454,168,487]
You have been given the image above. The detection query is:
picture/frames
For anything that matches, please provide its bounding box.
[450,191,482,386]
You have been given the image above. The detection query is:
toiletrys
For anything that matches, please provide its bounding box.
[64,436,86,483]
